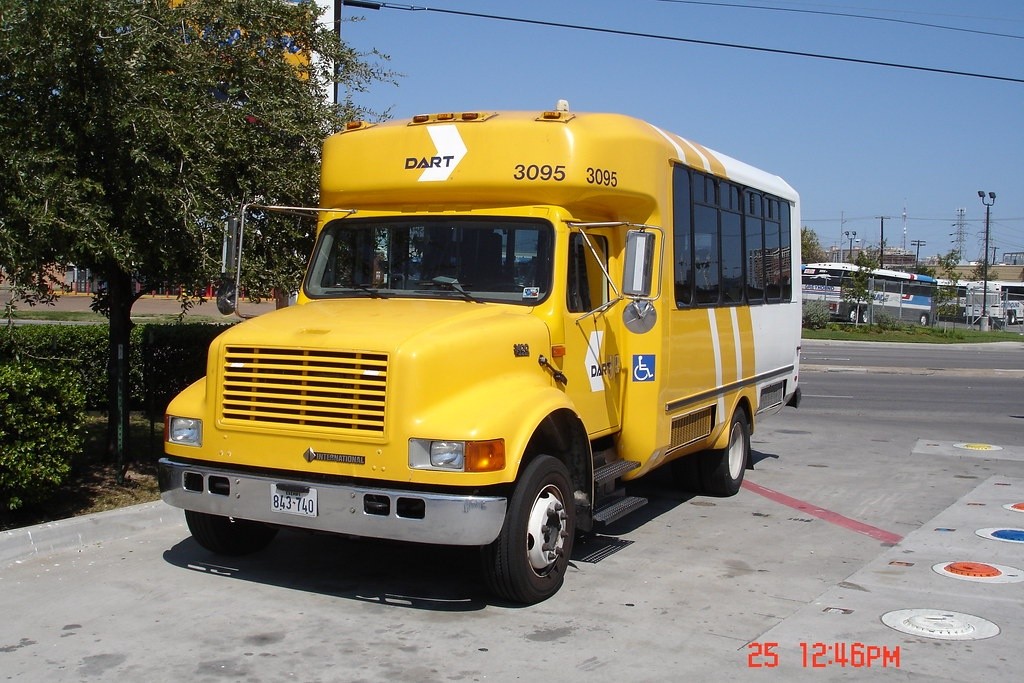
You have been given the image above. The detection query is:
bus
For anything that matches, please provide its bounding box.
[801,262,937,326]
[158,101,801,606]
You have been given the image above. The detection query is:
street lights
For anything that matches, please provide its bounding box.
[978,190,996,316]
[845,231,857,261]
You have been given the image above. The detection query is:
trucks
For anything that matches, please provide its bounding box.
[963,281,1002,324]
